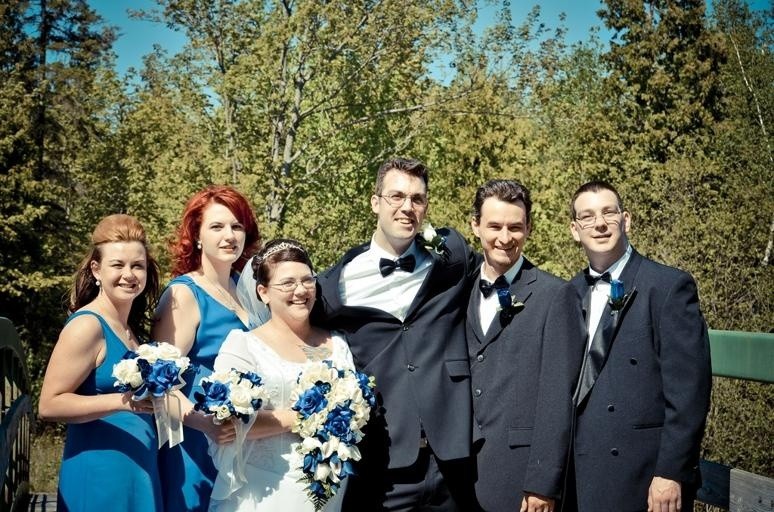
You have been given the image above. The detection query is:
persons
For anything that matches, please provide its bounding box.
[457,179,573,511]
[212,239,362,511]
[150,186,269,511]
[38,212,167,509]
[307,157,485,511]
[562,181,711,511]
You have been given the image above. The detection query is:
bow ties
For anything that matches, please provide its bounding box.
[379,254,416,276]
[479,274,509,298]
[586,271,613,286]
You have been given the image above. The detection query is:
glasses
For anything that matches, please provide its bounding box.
[270,275,316,292]
[576,211,619,228]
[379,191,428,208]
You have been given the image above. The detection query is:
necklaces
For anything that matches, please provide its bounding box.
[201,265,238,307]
[103,299,132,340]
